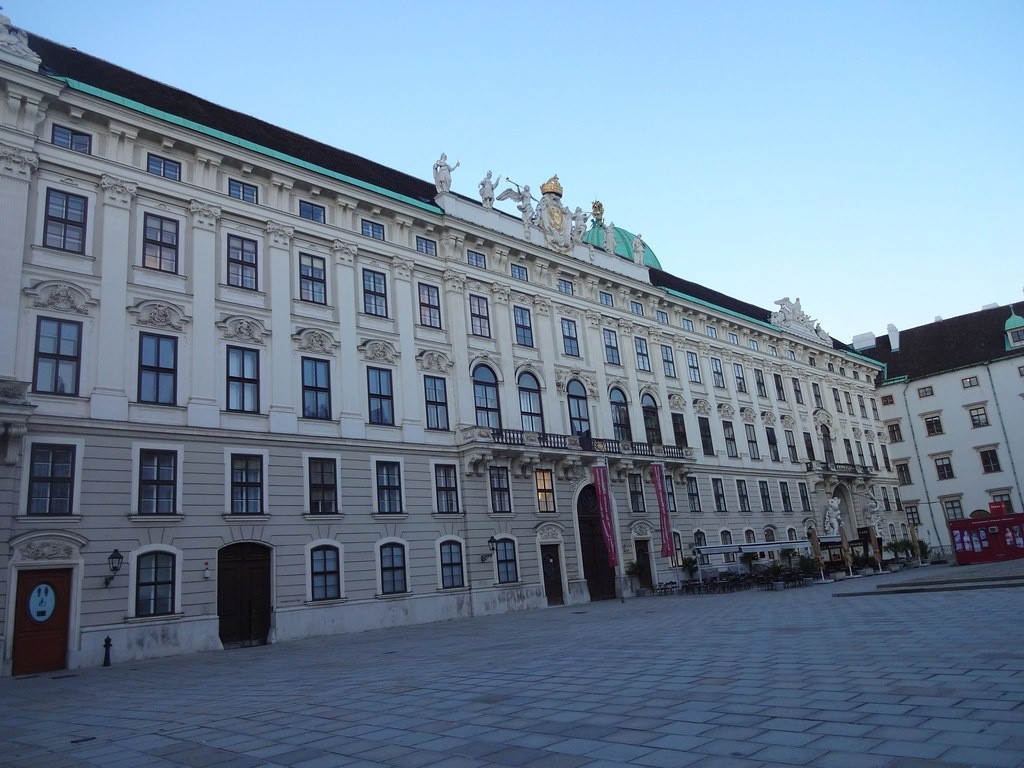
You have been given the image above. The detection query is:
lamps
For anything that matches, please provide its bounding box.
[104,549,124,588]
[481,536,498,562]
[927,530,931,536]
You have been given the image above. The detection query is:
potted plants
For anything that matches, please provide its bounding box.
[917,541,933,564]
[764,559,786,591]
[883,539,905,567]
[900,540,918,568]
[799,554,821,586]
[854,557,878,576]
[836,567,841,573]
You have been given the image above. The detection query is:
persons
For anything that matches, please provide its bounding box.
[431,153,647,268]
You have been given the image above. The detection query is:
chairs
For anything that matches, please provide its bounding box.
[681,558,906,596]
[650,581,679,596]
